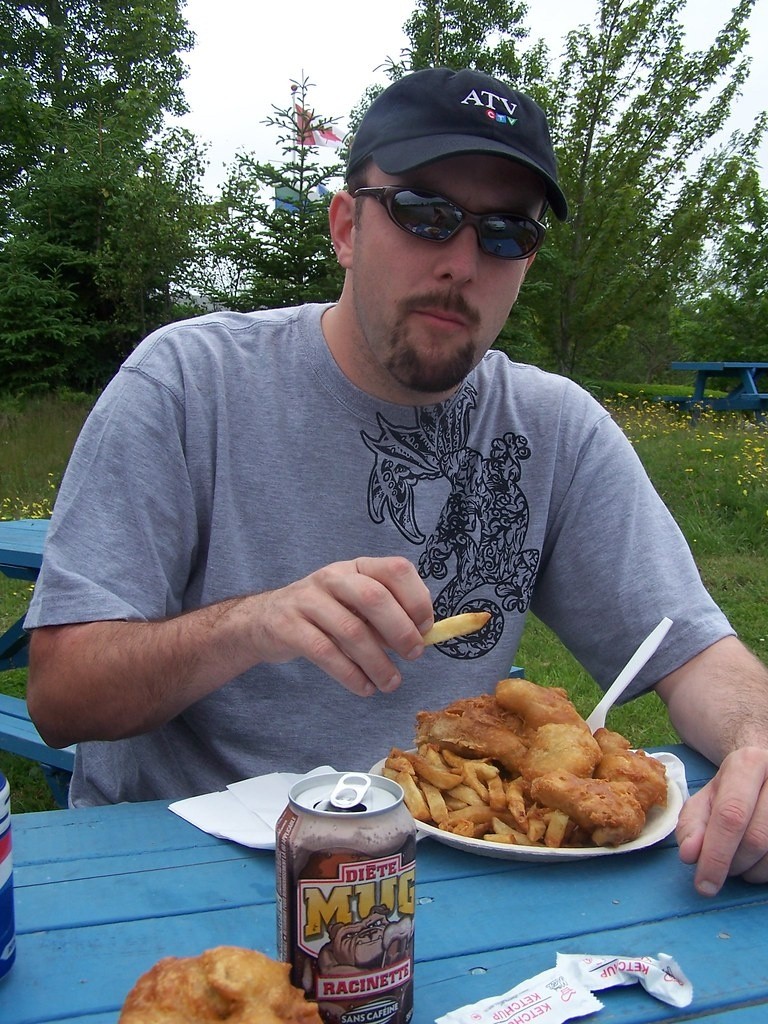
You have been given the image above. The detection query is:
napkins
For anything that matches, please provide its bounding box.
[168,766,339,851]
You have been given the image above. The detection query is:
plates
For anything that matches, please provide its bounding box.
[368,749,683,863]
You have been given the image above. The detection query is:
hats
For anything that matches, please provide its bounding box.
[343,67,568,224]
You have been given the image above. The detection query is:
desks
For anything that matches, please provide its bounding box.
[0,742,768,1024]
[671,359,768,428]
[0,515,52,670]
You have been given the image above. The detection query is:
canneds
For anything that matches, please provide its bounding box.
[274,772,417,1024]
[0,768,18,980]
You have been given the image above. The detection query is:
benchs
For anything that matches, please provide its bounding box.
[654,394,768,410]
[0,690,78,808]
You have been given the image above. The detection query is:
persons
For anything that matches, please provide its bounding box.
[22,70,767,898]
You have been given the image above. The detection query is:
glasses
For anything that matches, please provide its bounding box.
[352,184,548,260]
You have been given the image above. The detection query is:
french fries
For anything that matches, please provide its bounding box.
[421,612,492,644]
[382,745,586,851]
